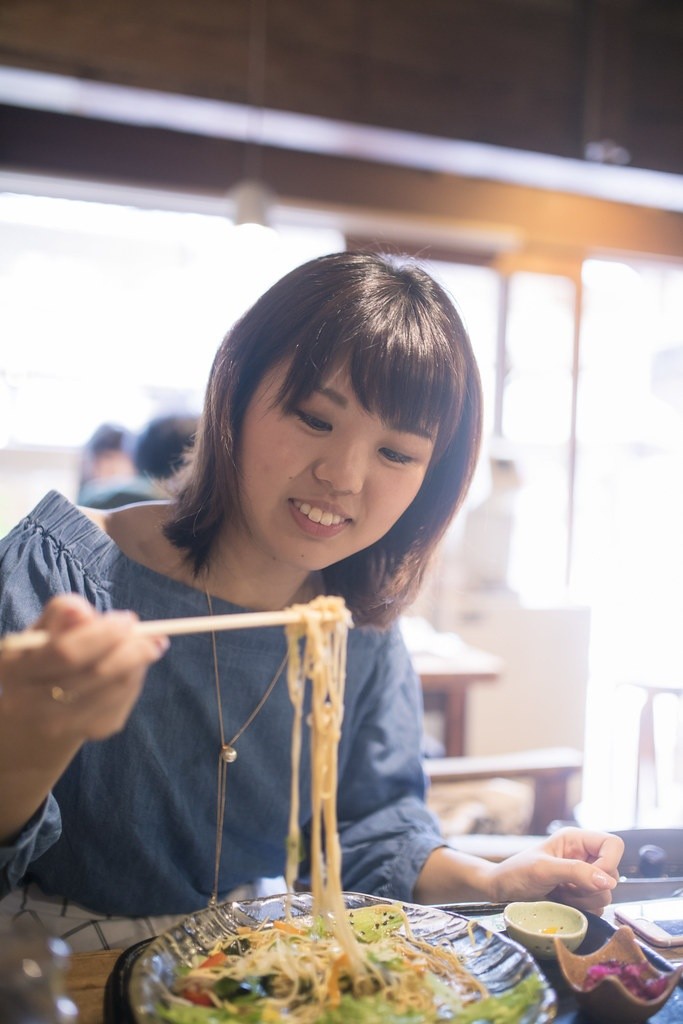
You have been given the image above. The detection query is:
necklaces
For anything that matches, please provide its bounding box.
[203,556,289,910]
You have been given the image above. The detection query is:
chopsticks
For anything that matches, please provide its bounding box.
[3,605,342,653]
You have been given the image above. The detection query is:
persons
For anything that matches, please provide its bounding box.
[1,249,625,950]
[74,412,204,510]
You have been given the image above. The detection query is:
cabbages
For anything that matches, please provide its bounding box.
[160,903,542,1024]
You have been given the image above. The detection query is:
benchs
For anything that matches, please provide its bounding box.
[423,748,582,836]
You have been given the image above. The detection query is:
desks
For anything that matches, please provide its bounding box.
[415,631,503,757]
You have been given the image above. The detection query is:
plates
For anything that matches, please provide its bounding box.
[129,892,557,1024]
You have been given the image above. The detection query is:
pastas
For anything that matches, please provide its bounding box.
[186,591,493,1024]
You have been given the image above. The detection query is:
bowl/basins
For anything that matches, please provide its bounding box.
[504,900,588,959]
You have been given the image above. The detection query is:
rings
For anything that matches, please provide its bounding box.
[52,683,76,703]
